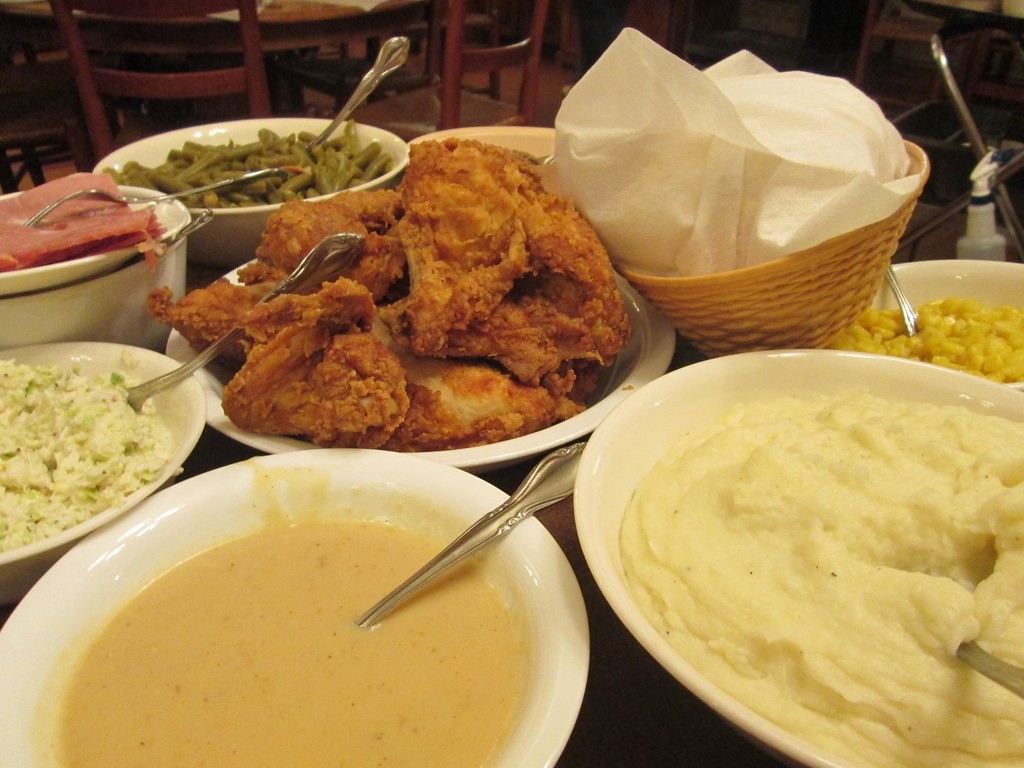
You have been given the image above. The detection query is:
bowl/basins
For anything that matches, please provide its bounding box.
[91,116,410,268]
[0,227,186,355]
[0,448,590,768]
[869,261,1023,388]
[0,341,210,609]
[573,348,1024,767]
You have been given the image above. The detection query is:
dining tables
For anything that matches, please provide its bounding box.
[2,203,1022,767]
[1,1,501,120]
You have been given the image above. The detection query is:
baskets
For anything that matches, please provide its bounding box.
[617,139,930,363]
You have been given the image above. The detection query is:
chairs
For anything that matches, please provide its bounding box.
[49,1,268,164]
[347,0,546,143]
[853,1,974,123]
[0,61,94,194]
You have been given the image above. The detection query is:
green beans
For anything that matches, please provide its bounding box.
[103,119,396,209]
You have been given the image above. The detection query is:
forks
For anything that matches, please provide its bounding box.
[22,163,304,228]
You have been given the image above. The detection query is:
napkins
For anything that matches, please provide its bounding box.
[540,26,922,269]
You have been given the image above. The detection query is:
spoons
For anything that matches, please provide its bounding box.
[304,36,408,154]
[883,265,917,334]
[124,232,365,413]
[357,439,586,633]
[954,640,1023,703]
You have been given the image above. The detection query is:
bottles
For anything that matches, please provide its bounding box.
[954,151,1005,264]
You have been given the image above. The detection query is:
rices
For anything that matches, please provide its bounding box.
[0,351,171,555]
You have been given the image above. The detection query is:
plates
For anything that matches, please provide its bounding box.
[404,125,568,167]
[0,182,190,296]
[167,254,680,476]
